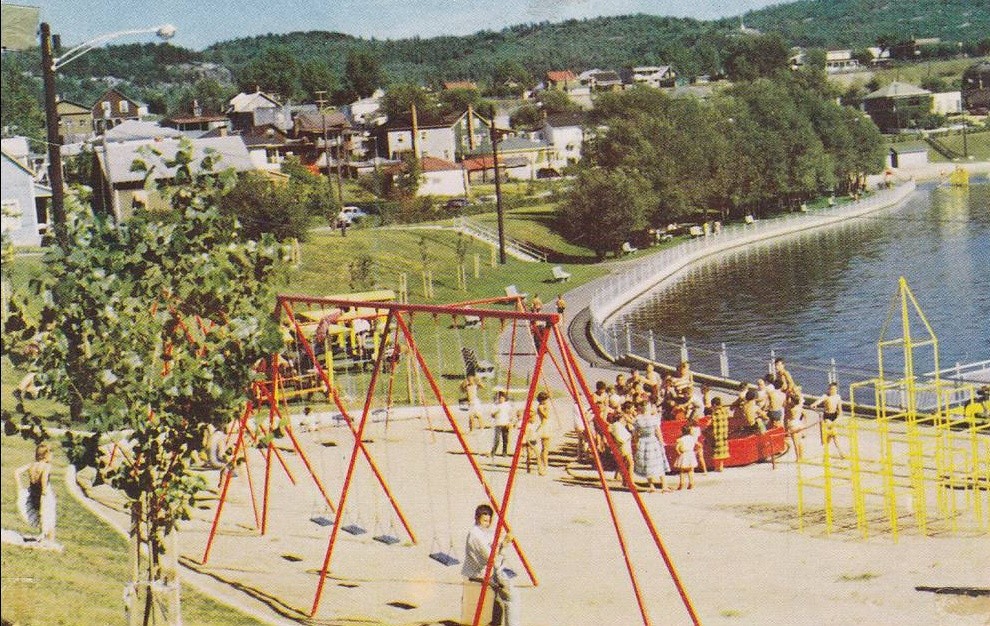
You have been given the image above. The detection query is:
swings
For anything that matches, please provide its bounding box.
[317,300,369,537]
[407,308,465,567]
[288,298,337,528]
[473,316,518,582]
[353,303,404,546]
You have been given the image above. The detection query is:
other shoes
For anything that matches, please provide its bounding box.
[645,483,695,491]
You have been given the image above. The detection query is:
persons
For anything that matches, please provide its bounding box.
[514,391,551,475]
[15,445,54,541]
[572,361,729,492]
[461,376,486,430]
[209,424,239,478]
[521,292,565,351]
[300,407,321,431]
[258,320,401,402]
[490,392,512,457]
[730,357,806,438]
[461,504,514,626]
[810,383,845,458]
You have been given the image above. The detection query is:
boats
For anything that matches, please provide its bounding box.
[932,385,990,431]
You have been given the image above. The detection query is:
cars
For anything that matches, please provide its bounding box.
[659,231,672,242]
[970,104,988,114]
[338,207,365,223]
[446,198,466,214]
[537,169,559,178]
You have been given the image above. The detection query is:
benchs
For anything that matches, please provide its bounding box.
[689,225,704,237]
[462,304,481,324]
[621,241,638,255]
[505,285,528,301]
[745,198,835,224]
[550,266,570,281]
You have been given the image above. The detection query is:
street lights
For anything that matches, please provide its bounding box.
[42,25,175,423]
[489,131,505,265]
